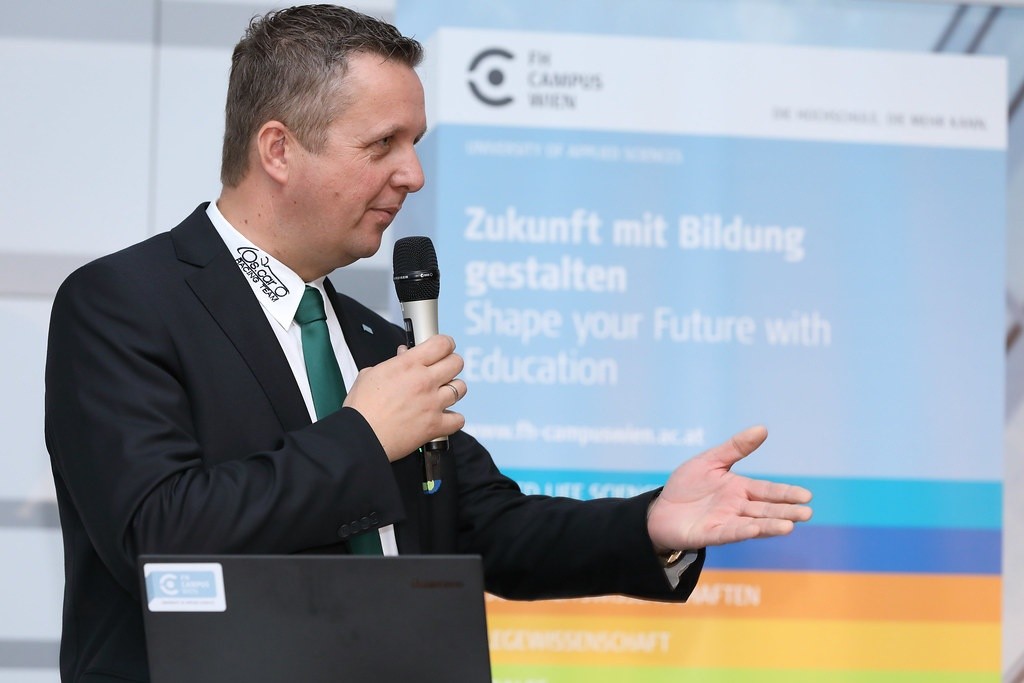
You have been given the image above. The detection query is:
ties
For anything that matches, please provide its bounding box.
[295,286,383,555]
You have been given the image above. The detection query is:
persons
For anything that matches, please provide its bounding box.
[45,4,813,683]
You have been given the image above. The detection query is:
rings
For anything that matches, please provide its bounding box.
[441,384,458,407]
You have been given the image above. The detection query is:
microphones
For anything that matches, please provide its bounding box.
[392,237,449,452]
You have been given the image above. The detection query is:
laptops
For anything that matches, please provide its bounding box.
[138,555,491,683]
